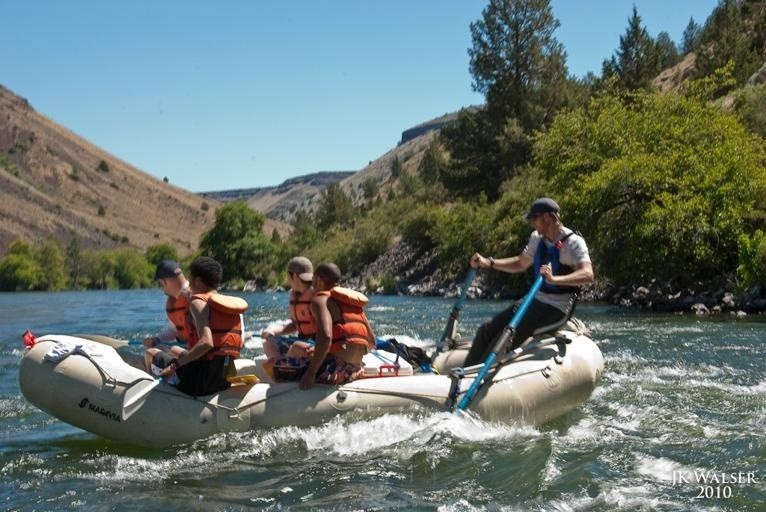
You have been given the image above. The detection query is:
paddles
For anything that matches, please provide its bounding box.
[71,333,190,350]
[227,374,260,385]
[244,331,316,345]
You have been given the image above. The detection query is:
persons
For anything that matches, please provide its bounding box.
[143,259,199,353]
[143,258,248,397]
[261,255,319,345]
[461,193,595,368]
[259,264,377,392]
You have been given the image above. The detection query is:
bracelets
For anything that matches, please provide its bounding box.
[174,360,179,369]
[169,361,176,372]
[488,255,495,269]
[151,338,156,347]
[155,336,160,344]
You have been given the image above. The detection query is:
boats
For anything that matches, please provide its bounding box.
[17,329,605,446]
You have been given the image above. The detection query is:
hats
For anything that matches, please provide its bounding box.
[150,259,181,284]
[522,198,560,220]
[287,256,314,281]
